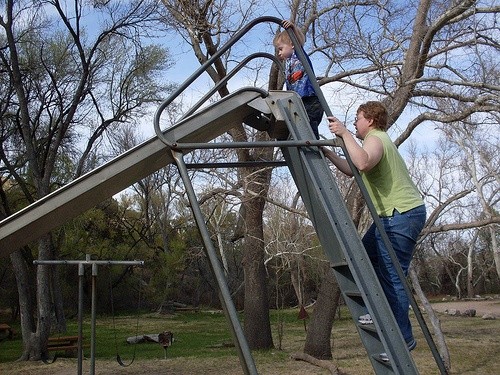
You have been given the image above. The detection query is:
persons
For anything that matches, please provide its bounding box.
[273,20,324,140]
[327,100,426,360]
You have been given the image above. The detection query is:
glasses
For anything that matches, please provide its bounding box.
[355,117,363,121]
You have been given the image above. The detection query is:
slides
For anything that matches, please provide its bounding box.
[0,86,269,256]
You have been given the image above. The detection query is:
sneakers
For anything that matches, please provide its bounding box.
[358,313,373,324]
[380,339,417,361]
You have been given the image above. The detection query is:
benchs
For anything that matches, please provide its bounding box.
[0,324,11,329]
[48,336,91,358]
